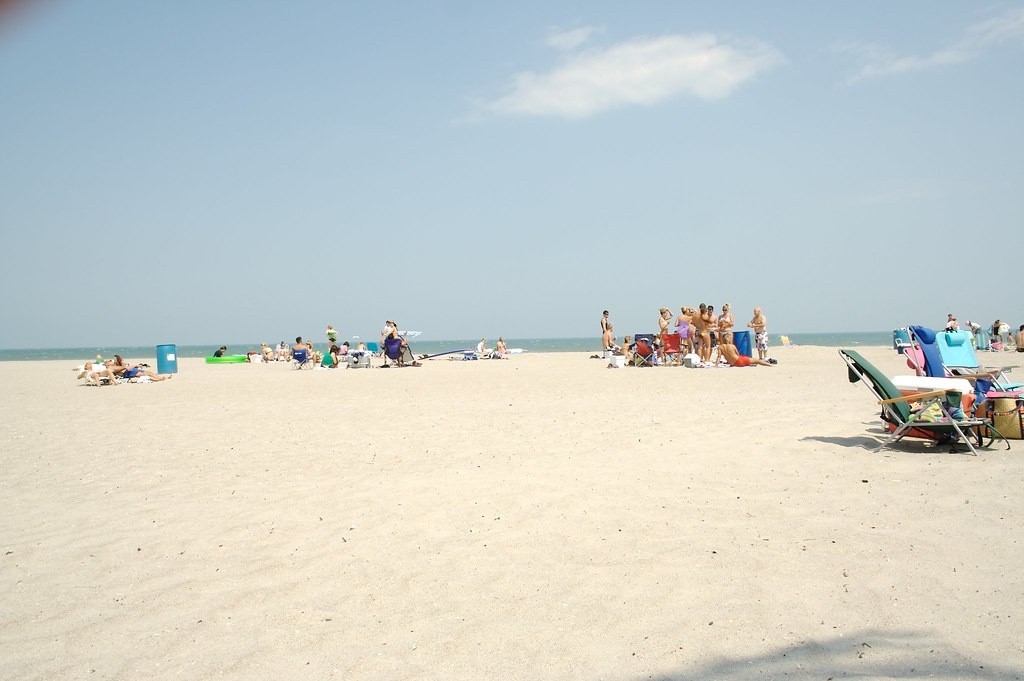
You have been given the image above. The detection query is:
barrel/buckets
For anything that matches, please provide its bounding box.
[733,331,752,358]
[894,330,909,349]
[157,344,177,374]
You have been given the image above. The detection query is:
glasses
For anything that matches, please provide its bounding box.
[708,309,712,310]
[605,314,609,315]
[723,310,727,311]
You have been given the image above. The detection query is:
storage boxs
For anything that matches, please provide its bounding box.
[603,350,612,359]
[897,343,912,354]
[611,356,626,368]
[888,375,975,441]
[684,353,701,367]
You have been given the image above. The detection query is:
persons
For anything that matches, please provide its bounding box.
[213,346,227,357]
[380,320,407,368]
[621,336,633,360]
[358,342,368,358]
[601,310,621,357]
[946,314,961,330]
[653,306,674,362]
[966,321,983,335]
[1007,332,1013,345]
[675,303,773,367]
[991,320,1005,343]
[77,355,172,388]
[320,325,350,368]
[497,337,506,355]
[1015,325,1024,353]
[477,336,490,354]
[261,337,316,368]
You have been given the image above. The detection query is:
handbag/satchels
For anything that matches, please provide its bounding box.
[967,398,1024,439]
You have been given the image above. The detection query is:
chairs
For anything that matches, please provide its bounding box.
[837,348,1011,456]
[290,346,314,369]
[104,360,150,384]
[381,339,408,367]
[661,332,683,366]
[903,324,1024,398]
[635,333,653,367]
[77,364,112,387]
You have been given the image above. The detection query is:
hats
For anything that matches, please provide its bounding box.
[387,319,394,323]
[965,321,970,326]
[660,308,668,315]
